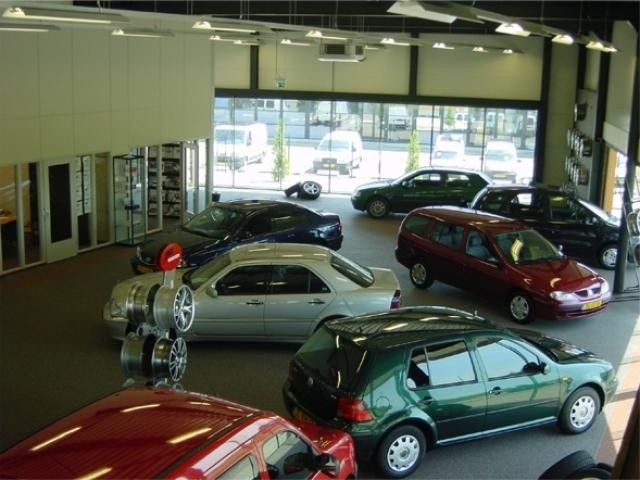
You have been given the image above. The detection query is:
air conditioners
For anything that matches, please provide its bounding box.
[317,43,366,62]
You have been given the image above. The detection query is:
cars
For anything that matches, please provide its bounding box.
[384,105,410,128]
[316,102,347,125]
[351,166,518,219]
[130,199,343,273]
[102,239,401,347]
[454,110,536,138]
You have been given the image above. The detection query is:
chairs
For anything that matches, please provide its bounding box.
[436,226,510,259]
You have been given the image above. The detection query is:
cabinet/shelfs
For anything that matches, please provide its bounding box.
[147,143,181,217]
[112,153,147,246]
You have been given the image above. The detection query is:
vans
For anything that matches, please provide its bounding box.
[484,141,518,182]
[215,122,267,170]
[432,134,464,167]
[313,130,363,175]
[395,204,611,323]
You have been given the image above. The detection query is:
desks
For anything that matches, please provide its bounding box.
[0,214,17,241]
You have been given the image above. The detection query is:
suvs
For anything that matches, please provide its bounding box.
[471,182,622,268]
[280,306,617,480]
[1,385,359,480]
